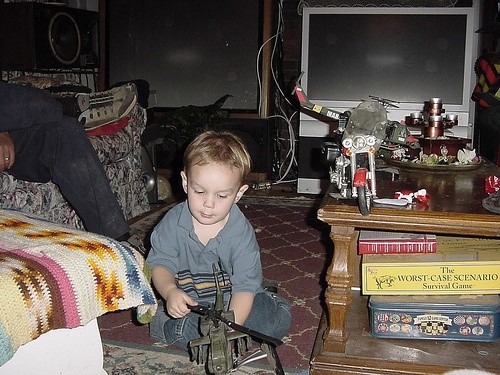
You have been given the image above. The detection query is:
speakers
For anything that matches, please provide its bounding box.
[0,2,101,69]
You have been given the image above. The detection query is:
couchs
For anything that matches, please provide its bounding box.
[1,76,159,374]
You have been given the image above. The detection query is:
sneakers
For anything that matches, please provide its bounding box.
[120,232,148,257]
[73,82,139,132]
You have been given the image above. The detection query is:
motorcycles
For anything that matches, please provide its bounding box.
[320,94,388,217]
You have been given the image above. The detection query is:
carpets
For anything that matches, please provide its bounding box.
[100,193,330,375]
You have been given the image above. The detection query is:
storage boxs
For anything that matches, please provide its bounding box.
[362,248,500,296]
[367,294,500,342]
[358,230,436,254]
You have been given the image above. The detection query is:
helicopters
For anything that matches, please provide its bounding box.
[189,262,283,375]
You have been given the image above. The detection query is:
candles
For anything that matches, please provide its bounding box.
[447,113,458,121]
[431,98,442,104]
[410,112,423,118]
[430,114,442,121]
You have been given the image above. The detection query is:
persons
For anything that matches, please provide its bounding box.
[146,130,292,351]
[0,81,149,261]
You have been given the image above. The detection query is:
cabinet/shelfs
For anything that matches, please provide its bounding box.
[297,137,475,194]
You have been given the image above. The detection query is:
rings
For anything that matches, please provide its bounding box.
[5,157,9,161]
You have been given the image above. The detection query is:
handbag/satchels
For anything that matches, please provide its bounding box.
[471,50,500,107]
[162,94,234,159]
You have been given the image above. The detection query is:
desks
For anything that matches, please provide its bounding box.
[309,157,500,375]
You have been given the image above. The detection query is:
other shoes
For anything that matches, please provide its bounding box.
[156,299,166,313]
[263,280,278,293]
